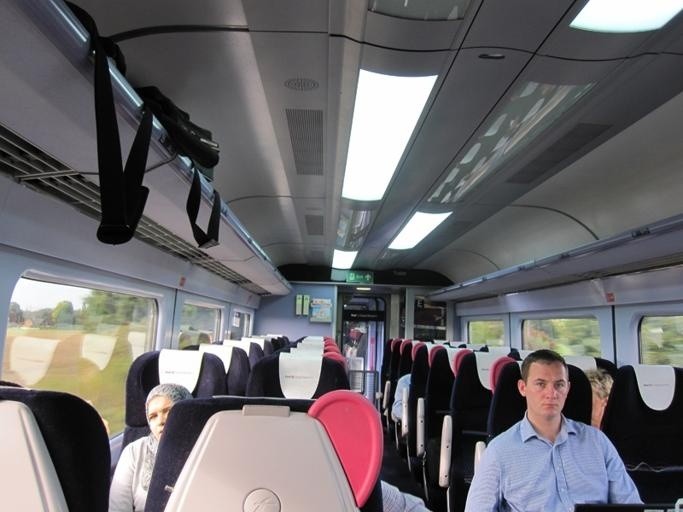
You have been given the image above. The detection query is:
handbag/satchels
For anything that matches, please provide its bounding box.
[132,86,220,169]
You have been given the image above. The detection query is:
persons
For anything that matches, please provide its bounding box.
[464,348,645,512]
[108,383,193,512]
[392,373,412,420]
[583,368,614,431]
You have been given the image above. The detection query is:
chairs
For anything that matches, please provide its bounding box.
[1,334,683,511]
